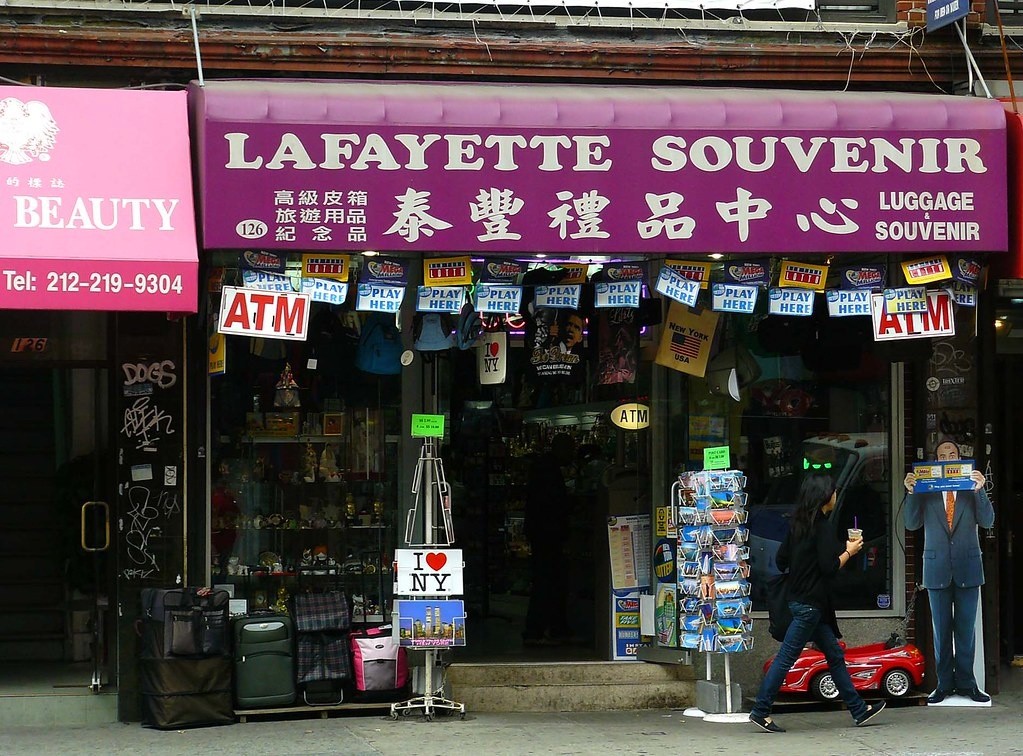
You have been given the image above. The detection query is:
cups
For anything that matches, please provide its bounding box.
[847,529,863,542]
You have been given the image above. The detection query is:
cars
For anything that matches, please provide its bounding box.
[764,632,925,703]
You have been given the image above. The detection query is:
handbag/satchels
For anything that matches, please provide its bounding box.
[764,573,843,642]
[349,625,408,692]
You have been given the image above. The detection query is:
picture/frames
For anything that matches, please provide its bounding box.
[349,407,386,481]
[321,411,344,437]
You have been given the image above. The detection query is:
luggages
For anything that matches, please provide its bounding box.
[229,609,296,707]
[135,585,234,731]
[291,590,351,706]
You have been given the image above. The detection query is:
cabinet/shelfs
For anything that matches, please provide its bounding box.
[220,434,399,623]
[461,399,647,622]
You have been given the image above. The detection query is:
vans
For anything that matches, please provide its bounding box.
[748,432,891,611]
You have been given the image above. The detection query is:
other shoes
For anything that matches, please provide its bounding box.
[551,636,573,648]
[523,639,550,646]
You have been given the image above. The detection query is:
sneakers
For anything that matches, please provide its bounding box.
[749,713,786,733]
[856,700,886,725]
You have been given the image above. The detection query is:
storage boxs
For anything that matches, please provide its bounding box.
[214,584,235,598]
[267,412,300,437]
[246,413,265,434]
[229,599,249,619]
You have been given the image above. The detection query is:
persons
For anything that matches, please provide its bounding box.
[902,441,997,702]
[748,471,887,734]
